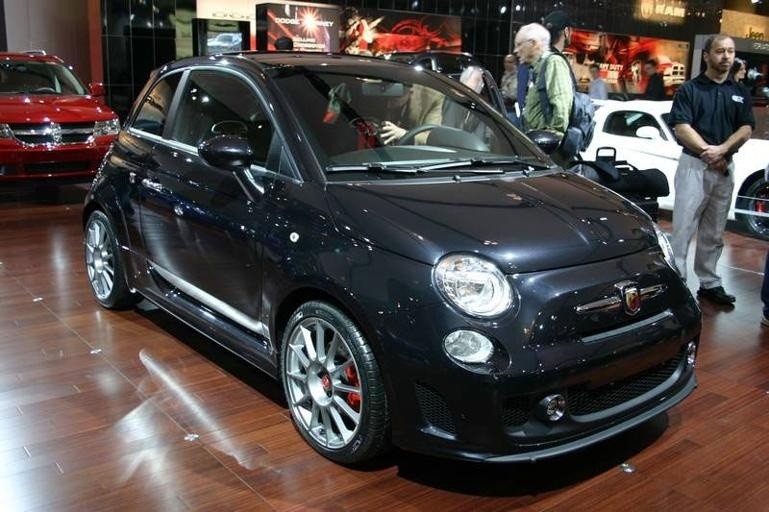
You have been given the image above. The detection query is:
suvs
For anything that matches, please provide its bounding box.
[0,48,123,196]
[205,32,241,51]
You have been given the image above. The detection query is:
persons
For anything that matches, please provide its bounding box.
[376,83,444,146]
[541,7,579,88]
[595,33,613,64]
[644,58,665,100]
[759,249,769,328]
[340,6,386,58]
[731,58,748,85]
[667,32,756,304]
[511,21,574,168]
[588,63,608,99]
[501,52,519,106]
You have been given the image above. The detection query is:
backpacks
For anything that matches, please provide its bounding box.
[538,49,597,160]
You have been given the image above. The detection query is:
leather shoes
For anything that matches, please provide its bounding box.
[696,285,736,304]
